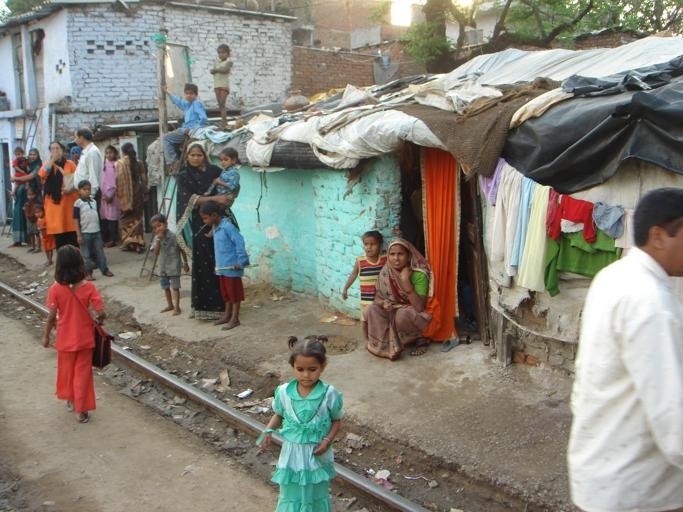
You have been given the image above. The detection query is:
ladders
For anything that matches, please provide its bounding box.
[2,108,42,238]
[140,139,188,281]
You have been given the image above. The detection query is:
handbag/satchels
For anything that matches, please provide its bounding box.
[92,324,115,371]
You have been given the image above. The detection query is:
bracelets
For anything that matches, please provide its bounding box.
[405,287,415,297]
[324,437,334,446]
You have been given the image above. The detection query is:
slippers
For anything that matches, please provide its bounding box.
[67,404,88,424]
[440,337,459,352]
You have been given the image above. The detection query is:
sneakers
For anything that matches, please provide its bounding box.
[106,271,113,276]
[86,275,96,281]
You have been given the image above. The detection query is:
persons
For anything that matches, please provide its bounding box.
[151,142,248,328]
[41,245,106,422]
[163,81,207,165]
[342,231,390,340]
[11,130,148,279]
[258,336,343,512]
[567,188,683,511]
[211,44,232,129]
[366,237,440,356]
